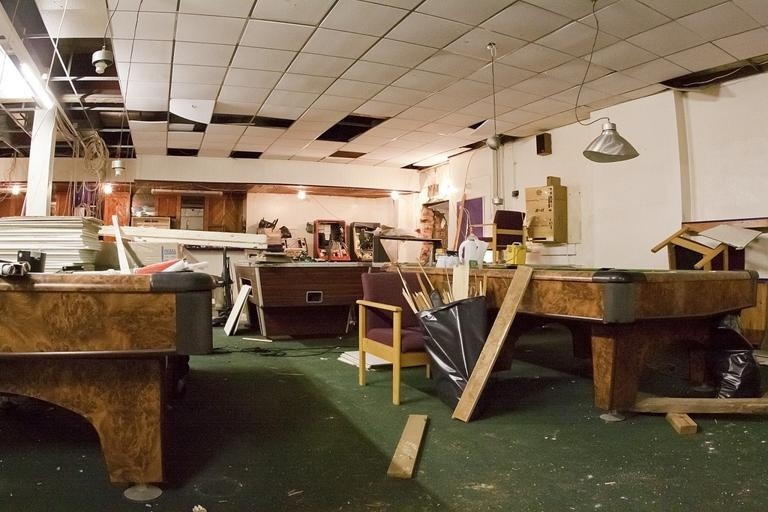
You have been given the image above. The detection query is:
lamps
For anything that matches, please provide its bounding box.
[110,0,143,175]
[492,148,503,206]
[150,189,223,196]
[91,0,119,74]
[573,0,640,162]
[484,43,501,152]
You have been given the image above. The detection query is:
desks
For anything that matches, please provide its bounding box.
[233,260,393,337]
[0,269,214,485]
[383,265,758,409]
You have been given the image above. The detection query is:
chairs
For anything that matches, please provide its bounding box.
[652,226,731,271]
[356,271,432,406]
[468,211,527,266]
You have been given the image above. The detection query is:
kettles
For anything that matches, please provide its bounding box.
[458,234,489,269]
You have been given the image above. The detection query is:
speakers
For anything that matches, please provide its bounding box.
[536,133,552,156]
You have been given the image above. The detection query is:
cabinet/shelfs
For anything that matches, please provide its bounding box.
[131,216,170,229]
[522,186,567,244]
